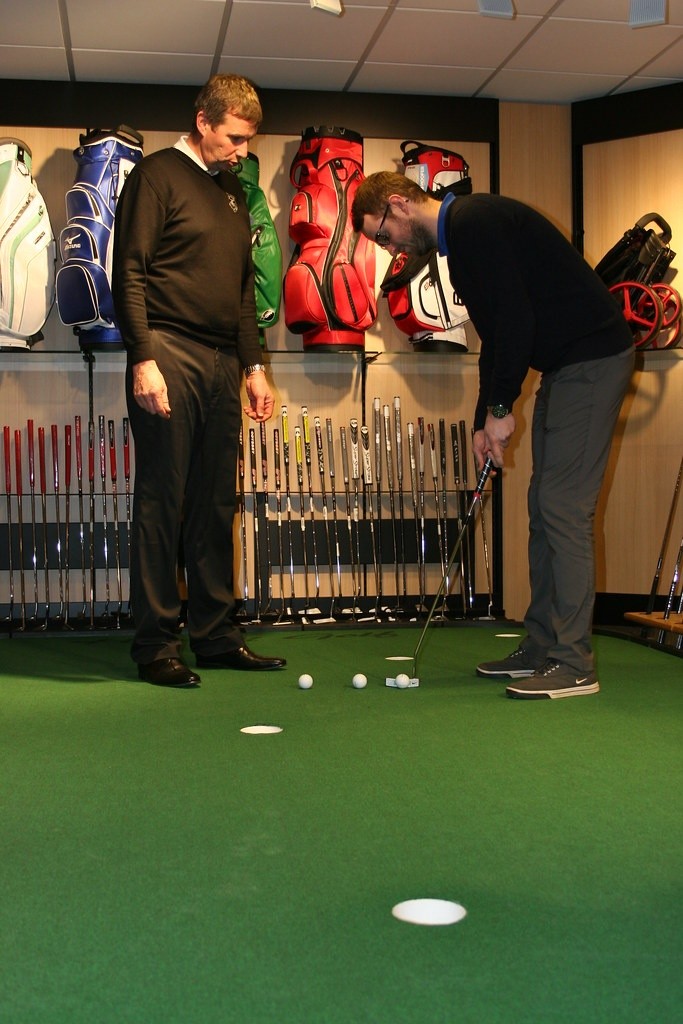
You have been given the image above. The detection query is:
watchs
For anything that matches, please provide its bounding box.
[487,404,513,419]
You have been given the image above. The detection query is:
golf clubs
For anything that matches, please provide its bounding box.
[385,456,494,688]
[641,456,683,650]
[235,395,496,626]
[0,415,132,633]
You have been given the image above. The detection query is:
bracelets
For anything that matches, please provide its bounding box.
[245,363,266,379]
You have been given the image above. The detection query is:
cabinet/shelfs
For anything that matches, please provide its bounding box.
[0,75,506,620]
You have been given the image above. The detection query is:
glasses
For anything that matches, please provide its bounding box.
[375,198,409,246]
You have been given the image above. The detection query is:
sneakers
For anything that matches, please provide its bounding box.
[505,659,599,700]
[475,649,553,679]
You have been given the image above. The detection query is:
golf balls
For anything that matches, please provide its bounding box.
[395,674,410,689]
[352,674,368,689]
[298,674,313,689]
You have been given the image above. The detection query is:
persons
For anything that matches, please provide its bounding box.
[113,74,288,685]
[352,171,636,699]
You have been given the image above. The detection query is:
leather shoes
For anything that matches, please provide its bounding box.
[195,645,286,671]
[137,658,201,686]
